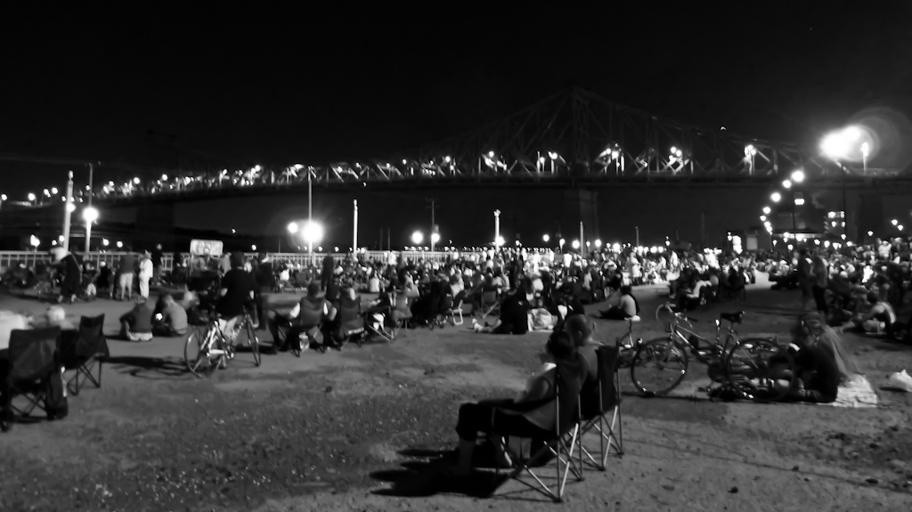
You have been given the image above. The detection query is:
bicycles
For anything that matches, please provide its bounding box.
[182,295,263,377]
[626,296,801,404]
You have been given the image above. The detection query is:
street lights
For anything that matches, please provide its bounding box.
[753,128,857,251]
[79,204,99,261]
[302,221,326,264]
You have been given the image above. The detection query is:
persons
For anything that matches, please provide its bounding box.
[50,243,911,478]
